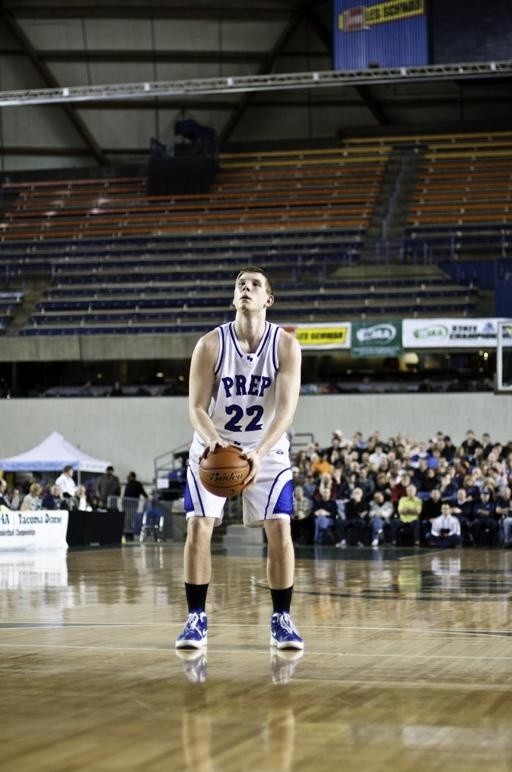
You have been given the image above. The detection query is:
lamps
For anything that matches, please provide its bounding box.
[61,86,72,97]
[311,71,325,81]
[488,61,503,72]
[143,82,153,92]
[226,76,239,87]
[399,65,413,76]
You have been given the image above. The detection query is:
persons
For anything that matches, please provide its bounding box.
[295,551,509,617]
[0,443,189,543]
[173,649,305,772]
[173,267,305,654]
[283,427,512,553]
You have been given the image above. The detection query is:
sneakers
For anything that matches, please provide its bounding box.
[175,609,207,649]
[270,611,304,650]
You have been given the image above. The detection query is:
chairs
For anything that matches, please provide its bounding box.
[137,508,165,542]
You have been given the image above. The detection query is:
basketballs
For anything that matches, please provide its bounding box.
[198,446,253,497]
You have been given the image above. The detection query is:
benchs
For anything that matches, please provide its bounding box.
[1,128,512,317]
